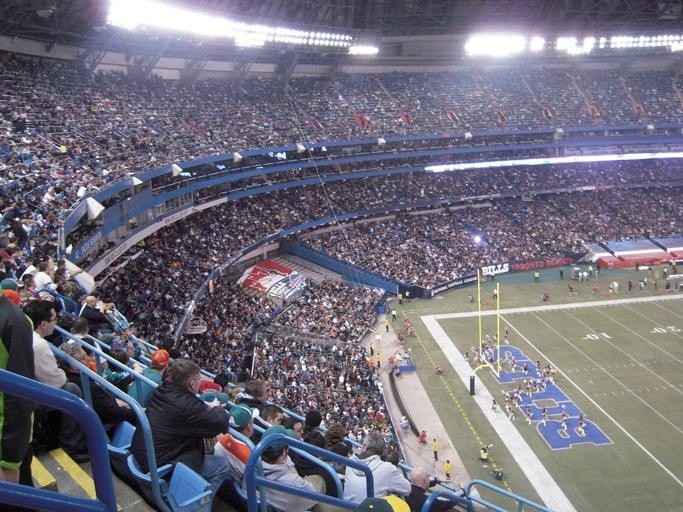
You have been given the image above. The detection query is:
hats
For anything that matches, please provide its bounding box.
[358,495,411,512]
[261,425,296,452]
[334,440,352,456]
[0,278,24,289]
[228,404,260,429]
[198,389,229,405]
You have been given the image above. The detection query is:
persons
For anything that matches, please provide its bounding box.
[1,52,682,511]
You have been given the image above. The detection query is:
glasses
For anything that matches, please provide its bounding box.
[44,317,59,321]
